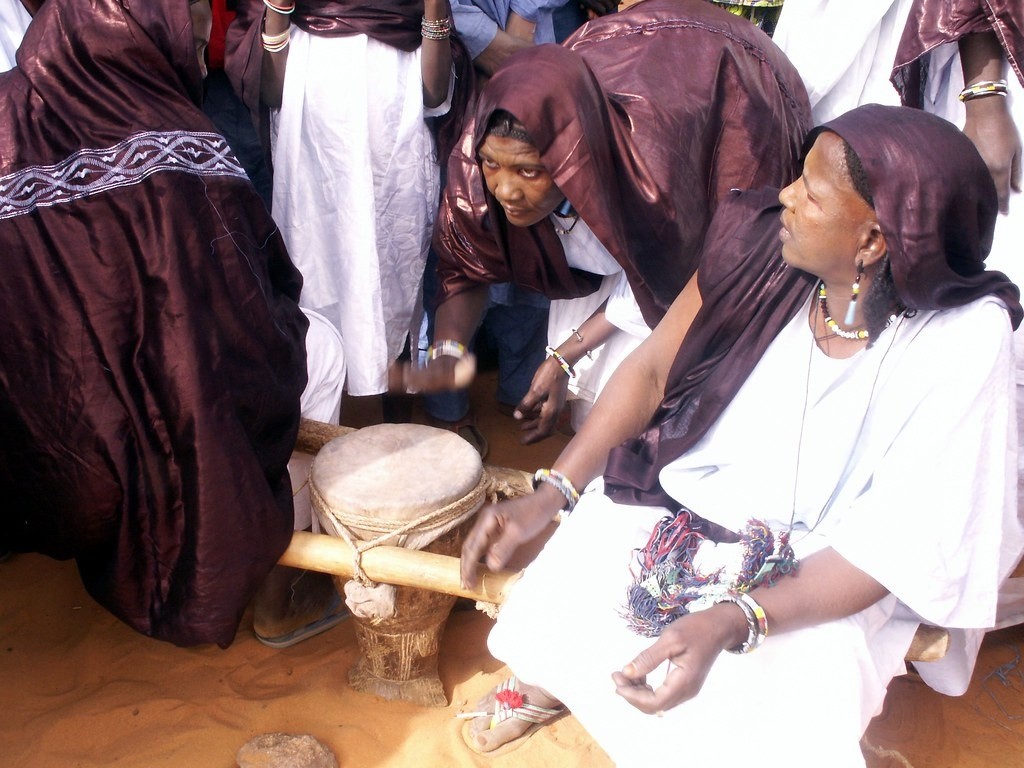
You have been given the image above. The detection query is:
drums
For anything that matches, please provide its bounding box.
[305,424,493,709]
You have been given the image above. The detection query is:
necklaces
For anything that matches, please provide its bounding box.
[545,205,582,236]
[814,280,898,339]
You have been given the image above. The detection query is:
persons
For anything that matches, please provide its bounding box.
[222,0,476,430]
[379,1,824,509]
[446,0,619,119]
[763,0,1024,304]
[2,0,359,647]
[454,102,1024,768]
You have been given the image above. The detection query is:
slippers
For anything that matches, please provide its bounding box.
[255,591,354,649]
[461,675,571,757]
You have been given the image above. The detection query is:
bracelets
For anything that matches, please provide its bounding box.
[544,345,577,380]
[958,79,1009,102]
[256,23,292,54]
[726,589,767,651]
[426,337,469,363]
[263,0,296,15]
[420,14,453,43]
[267,0,296,10]
[531,474,575,513]
[570,328,593,362]
[698,594,756,655]
[533,468,580,505]
